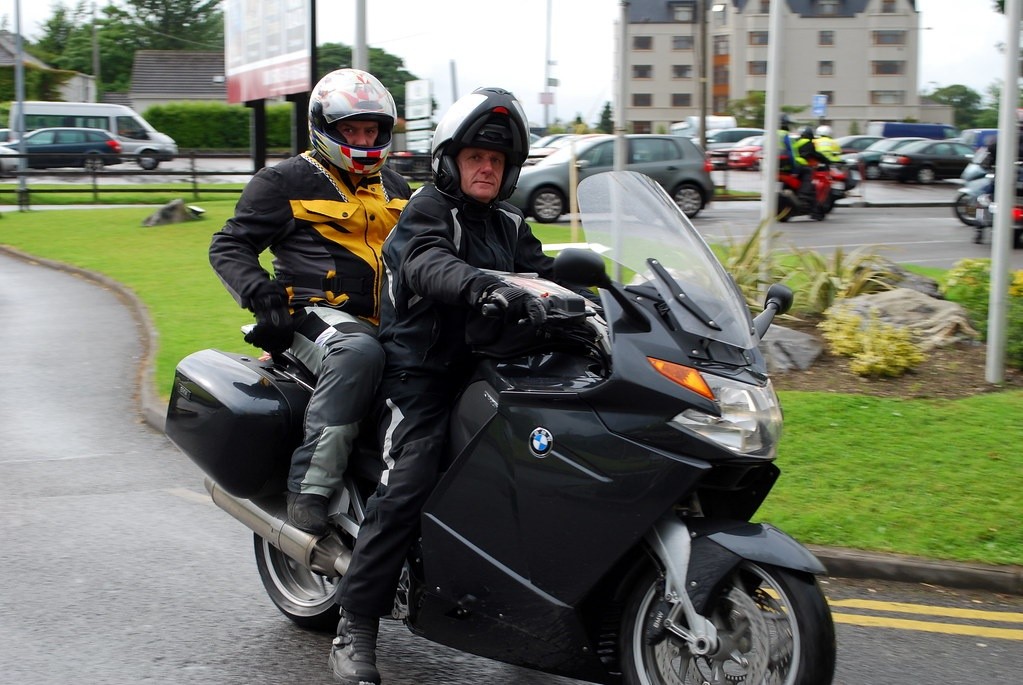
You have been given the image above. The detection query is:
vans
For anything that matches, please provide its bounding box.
[8,102,180,170]
[962,128,997,147]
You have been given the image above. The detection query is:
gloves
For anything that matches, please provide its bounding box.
[244,305,295,353]
[479,281,548,329]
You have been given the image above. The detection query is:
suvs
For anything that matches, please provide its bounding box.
[2,127,122,171]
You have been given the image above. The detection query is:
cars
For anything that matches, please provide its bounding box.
[696,127,763,169]
[508,132,714,223]
[834,136,977,182]
[0,145,19,178]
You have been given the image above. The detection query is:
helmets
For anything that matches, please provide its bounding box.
[308,68,399,175]
[796,125,813,138]
[816,125,834,137]
[430,87,530,211]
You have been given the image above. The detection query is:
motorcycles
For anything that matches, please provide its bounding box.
[162,167,837,685]
[954,148,1023,247]
[777,160,848,223]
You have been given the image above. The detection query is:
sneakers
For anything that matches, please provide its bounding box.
[287,493,330,535]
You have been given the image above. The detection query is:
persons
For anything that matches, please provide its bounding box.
[208,67,412,536]
[328,91,607,685]
[778,111,846,223]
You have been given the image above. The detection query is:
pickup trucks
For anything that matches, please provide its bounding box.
[387,149,431,181]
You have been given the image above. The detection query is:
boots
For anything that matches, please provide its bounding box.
[328,606,382,685]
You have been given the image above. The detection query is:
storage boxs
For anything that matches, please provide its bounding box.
[164,349,310,500]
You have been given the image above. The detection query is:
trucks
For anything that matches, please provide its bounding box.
[671,116,736,140]
[867,122,959,138]
